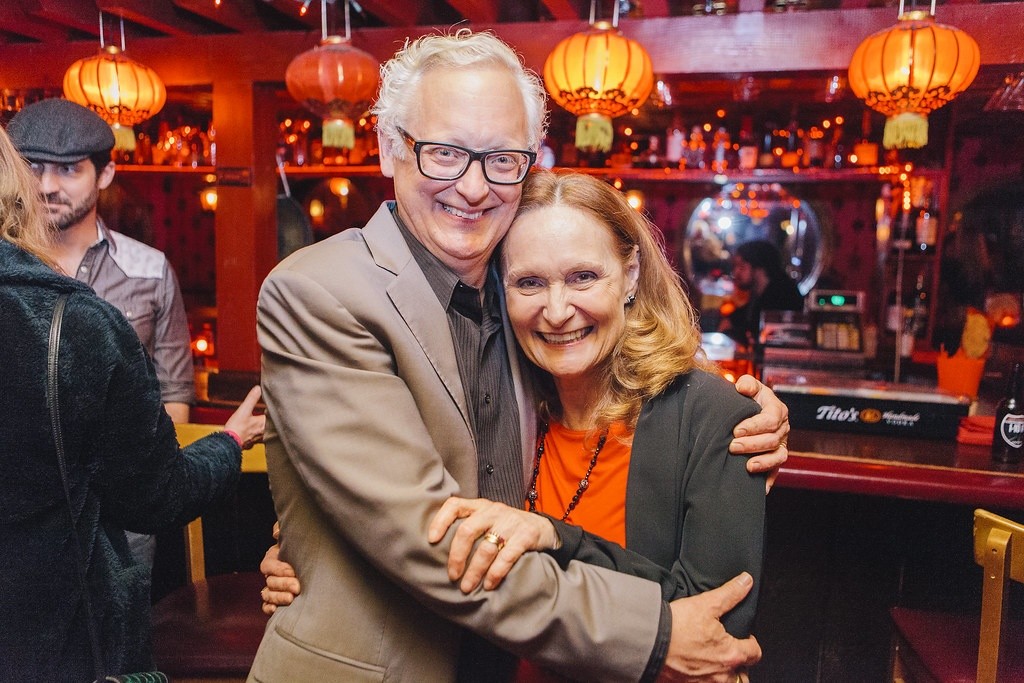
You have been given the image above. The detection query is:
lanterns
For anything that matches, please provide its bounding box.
[284,34,384,148]
[848,12,979,150]
[62,44,167,152]
[543,22,655,152]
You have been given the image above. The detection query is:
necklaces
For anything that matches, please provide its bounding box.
[526,412,611,523]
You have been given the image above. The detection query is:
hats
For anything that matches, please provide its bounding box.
[5,97,115,164]
[722,241,788,276]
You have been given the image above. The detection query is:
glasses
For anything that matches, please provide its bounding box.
[395,125,538,185]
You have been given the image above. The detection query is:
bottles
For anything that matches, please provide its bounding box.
[108,111,383,165]
[991,361,1024,463]
[889,268,927,351]
[816,312,860,352]
[187,322,219,388]
[876,180,939,247]
[548,104,878,169]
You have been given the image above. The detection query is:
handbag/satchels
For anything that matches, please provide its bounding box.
[92,671,172,683]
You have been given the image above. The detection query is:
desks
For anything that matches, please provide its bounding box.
[771,429,1023,510]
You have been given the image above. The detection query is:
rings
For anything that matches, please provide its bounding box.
[736,671,743,683]
[483,535,504,552]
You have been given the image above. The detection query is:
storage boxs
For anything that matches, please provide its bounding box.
[772,383,969,444]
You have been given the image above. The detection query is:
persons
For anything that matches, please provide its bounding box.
[244,30,791,683]
[0,123,267,683]
[719,238,804,346]
[4,99,194,626]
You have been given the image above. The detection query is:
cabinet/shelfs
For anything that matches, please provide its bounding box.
[0,1,1023,365]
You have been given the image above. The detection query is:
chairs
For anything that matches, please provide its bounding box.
[887,509,1024,683]
[151,421,269,683]
